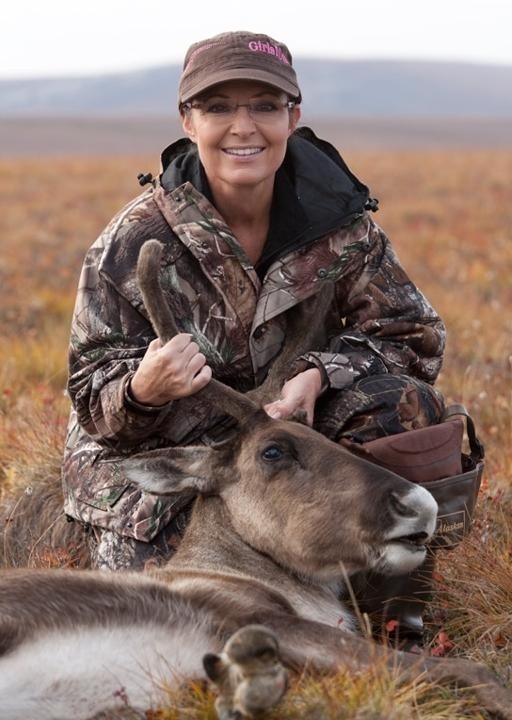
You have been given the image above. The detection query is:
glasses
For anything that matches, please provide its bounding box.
[184,101,297,124]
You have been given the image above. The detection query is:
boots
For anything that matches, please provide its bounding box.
[342,404,486,640]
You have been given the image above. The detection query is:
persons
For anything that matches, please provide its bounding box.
[57,29,448,646]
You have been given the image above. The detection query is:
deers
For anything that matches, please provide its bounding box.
[0,238,512,720]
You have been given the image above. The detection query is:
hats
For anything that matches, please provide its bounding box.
[178,32,301,107]
